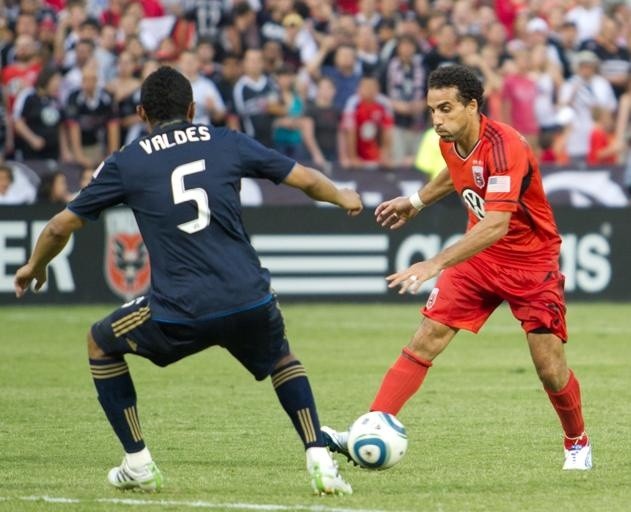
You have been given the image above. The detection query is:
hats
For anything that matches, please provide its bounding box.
[527,16,551,34]
[573,49,601,73]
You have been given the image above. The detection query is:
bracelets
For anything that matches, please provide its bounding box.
[408,190,427,213]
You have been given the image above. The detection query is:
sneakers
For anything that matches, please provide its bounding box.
[307,459,355,496]
[320,425,360,466]
[107,458,164,494]
[561,439,594,472]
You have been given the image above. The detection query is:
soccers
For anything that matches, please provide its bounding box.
[348,411,408,469]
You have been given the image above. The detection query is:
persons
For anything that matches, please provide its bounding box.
[1,0,631,205]
[320,65,594,471]
[12,64,364,496]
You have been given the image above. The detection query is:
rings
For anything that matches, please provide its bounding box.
[410,275,419,281]
